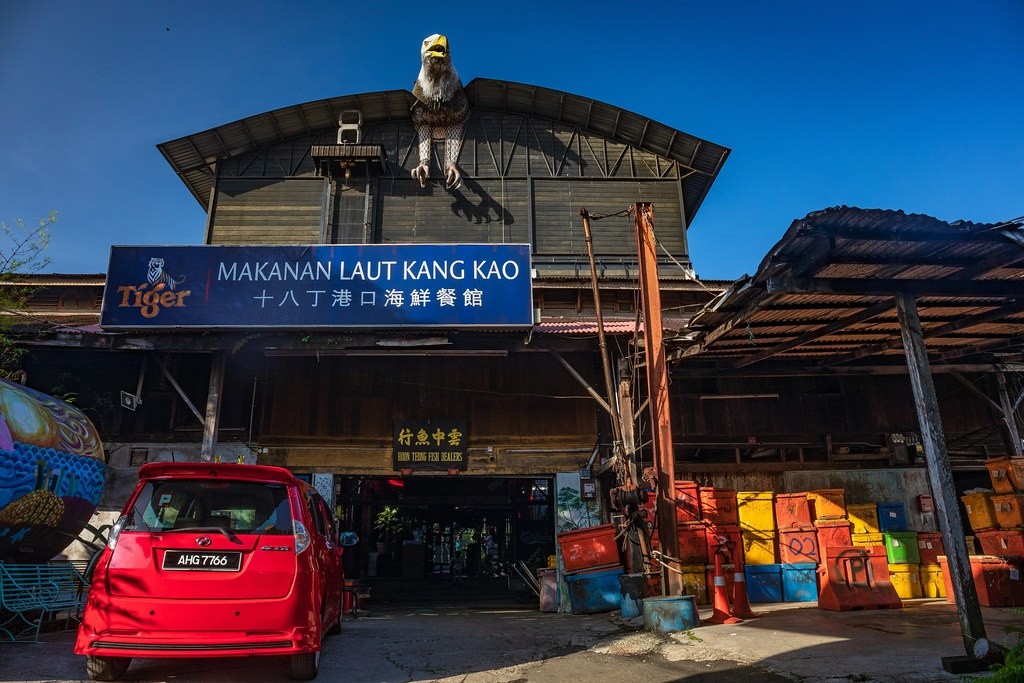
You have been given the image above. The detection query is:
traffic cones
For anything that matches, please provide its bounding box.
[728,552,764,620]
[701,553,742,624]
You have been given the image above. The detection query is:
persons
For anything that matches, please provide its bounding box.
[481,528,495,576]
[448,552,466,585]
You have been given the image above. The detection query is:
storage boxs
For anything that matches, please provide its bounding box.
[553,455,1024,615]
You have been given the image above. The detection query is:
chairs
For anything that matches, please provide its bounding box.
[173,515,284,536]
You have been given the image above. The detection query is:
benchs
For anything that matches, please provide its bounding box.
[0,560,92,643]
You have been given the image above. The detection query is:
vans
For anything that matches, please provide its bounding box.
[72,459,346,683]
